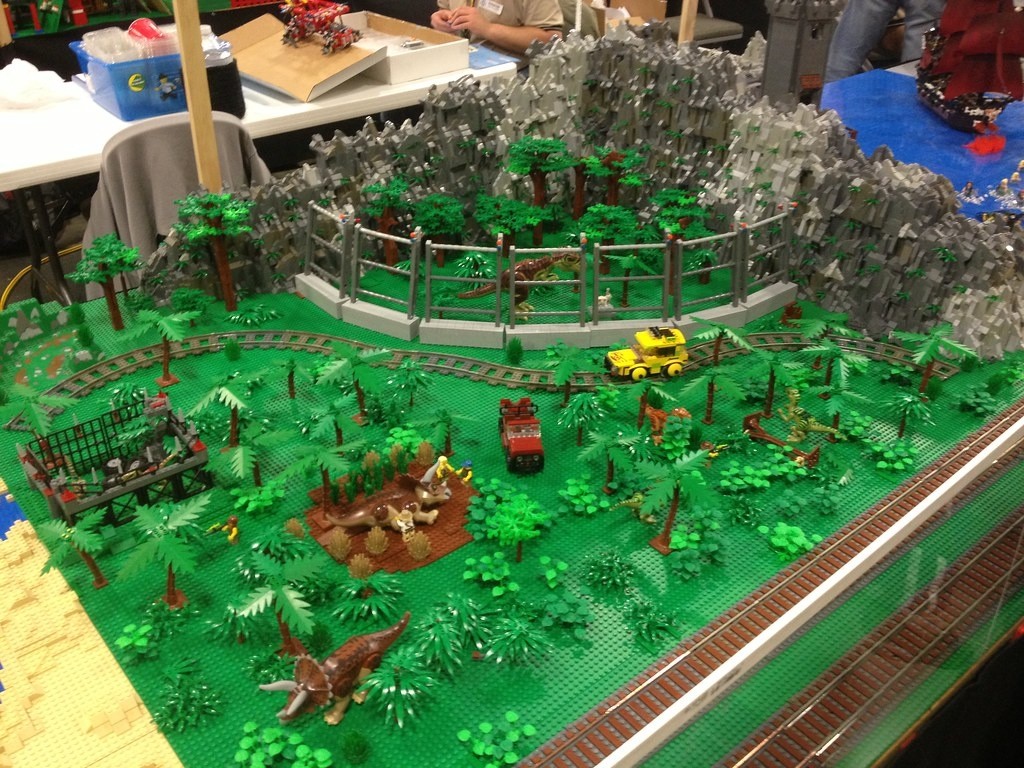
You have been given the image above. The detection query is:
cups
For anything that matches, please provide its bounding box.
[129,18,167,42]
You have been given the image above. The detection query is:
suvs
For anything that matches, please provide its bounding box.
[496,396,546,473]
[603,325,690,383]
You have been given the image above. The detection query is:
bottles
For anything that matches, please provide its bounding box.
[199,24,233,69]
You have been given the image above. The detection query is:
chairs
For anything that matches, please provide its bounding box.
[609,0,744,46]
[81,109,271,301]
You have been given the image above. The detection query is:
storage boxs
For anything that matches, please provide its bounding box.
[67,41,187,122]
[217,10,470,102]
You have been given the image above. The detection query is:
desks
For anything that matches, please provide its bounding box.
[0,61,517,304]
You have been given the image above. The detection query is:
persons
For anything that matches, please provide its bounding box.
[952,98,1007,132]
[824,0,948,85]
[558,0,601,41]
[430,0,564,79]
